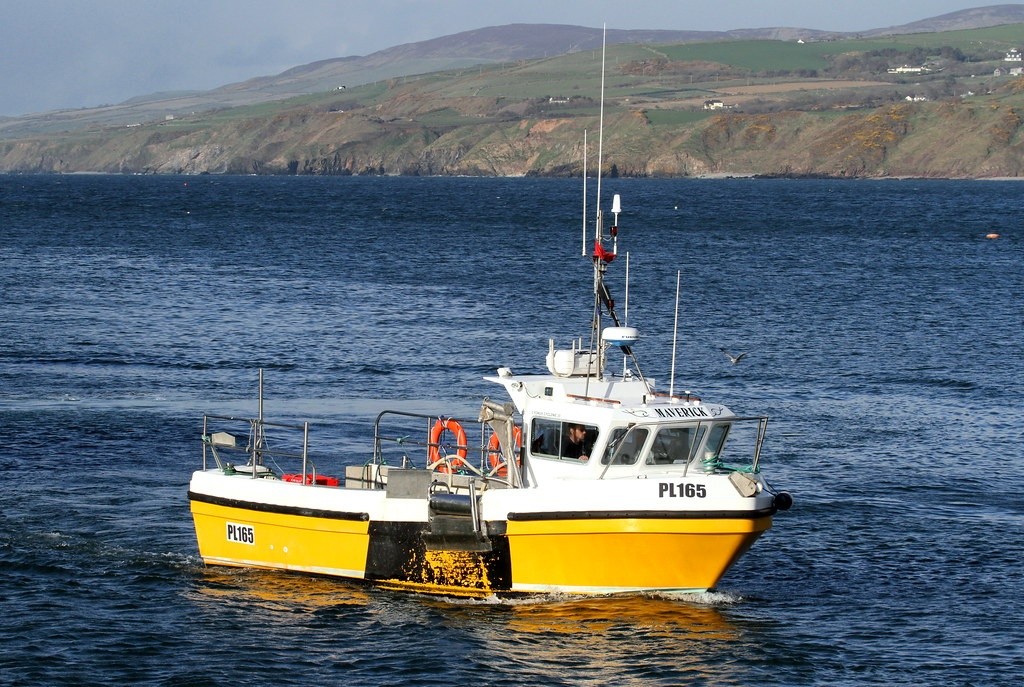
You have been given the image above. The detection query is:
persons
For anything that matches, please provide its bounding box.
[625,429,656,466]
[556,422,589,462]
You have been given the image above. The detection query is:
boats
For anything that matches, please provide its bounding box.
[186,24,792,596]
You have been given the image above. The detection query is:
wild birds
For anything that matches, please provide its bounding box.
[719,346,761,364]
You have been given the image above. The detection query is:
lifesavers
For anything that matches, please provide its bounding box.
[428,420,469,476]
[489,426,523,477]
